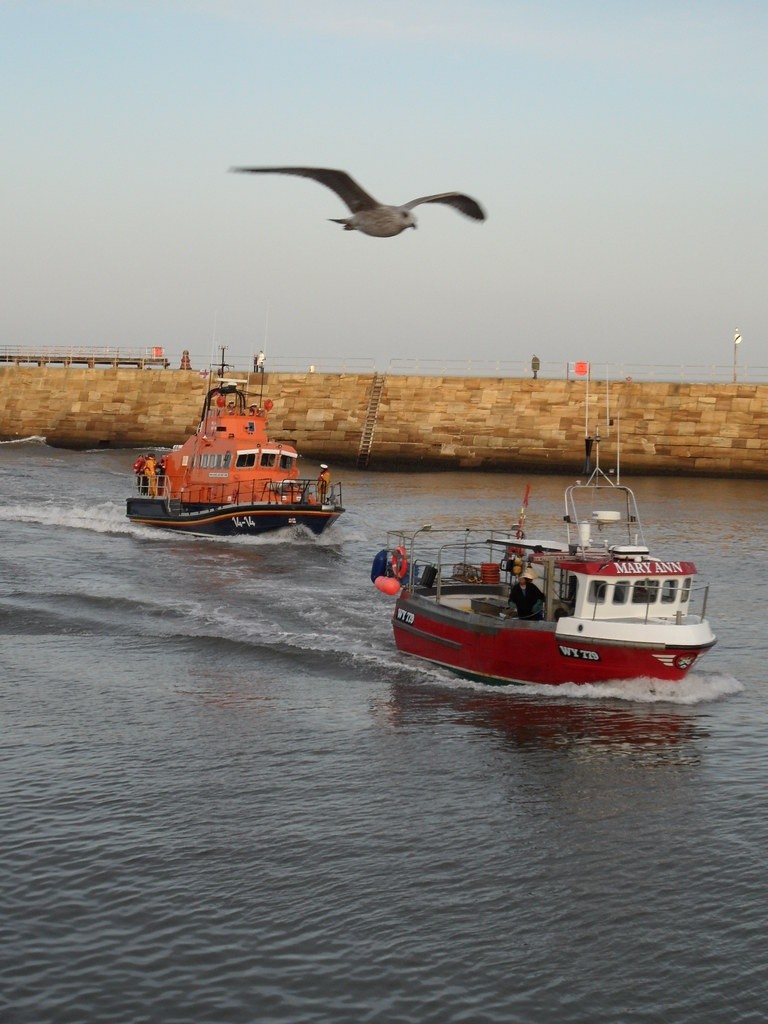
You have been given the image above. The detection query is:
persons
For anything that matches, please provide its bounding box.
[133,453,164,497]
[256,350,265,372]
[318,463,331,503]
[253,352,258,372]
[508,573,545,620]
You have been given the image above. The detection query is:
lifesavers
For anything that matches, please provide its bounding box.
[575,361,589,375]
[392,546,407,577]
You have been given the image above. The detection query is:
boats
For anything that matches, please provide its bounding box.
[388,363,717,688]
[125,345,345,540]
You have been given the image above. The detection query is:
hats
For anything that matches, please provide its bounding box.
[320,464,328,469]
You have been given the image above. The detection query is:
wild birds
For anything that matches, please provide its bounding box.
[231,166,487,238]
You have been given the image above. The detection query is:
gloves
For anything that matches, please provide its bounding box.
[532,599,542,613]
[509,601,517,609]
